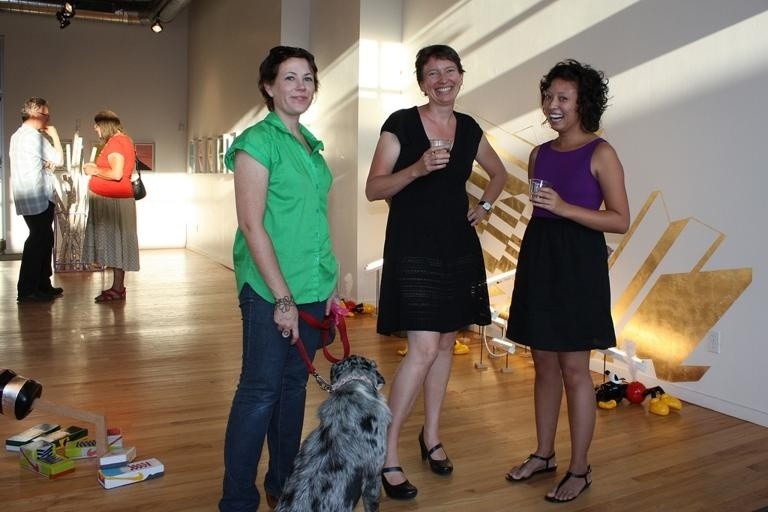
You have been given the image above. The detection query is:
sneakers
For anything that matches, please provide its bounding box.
[18,287,63,303]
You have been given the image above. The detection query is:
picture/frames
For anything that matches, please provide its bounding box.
[134,142,155,172]
[51,141,85,172]
[90,141,105,165]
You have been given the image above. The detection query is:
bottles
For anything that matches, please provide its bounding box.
[106,427,121,447]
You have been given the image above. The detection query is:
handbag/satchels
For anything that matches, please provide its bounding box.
[132,179,146,200]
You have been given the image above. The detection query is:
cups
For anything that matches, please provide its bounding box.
[527,177,553,203]
[429,139,452,156]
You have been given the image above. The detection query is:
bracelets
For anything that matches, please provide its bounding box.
[275,296,295,313]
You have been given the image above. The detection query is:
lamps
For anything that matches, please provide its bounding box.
[150,14,164,34]
[55,1,76,31]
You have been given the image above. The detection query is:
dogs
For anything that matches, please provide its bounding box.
[274,354,393,512]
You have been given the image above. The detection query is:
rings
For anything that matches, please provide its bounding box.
[281,327,291,339]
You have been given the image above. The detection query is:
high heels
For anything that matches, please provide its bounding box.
[419,426,453,476]
[381,467,418,499]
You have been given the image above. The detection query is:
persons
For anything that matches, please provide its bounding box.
[365,44,508,501]
[80,109,140,302]
[504,59,631,504]
[218,45,342,511]
[8,96,65,303]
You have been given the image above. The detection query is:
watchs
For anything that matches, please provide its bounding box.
[478,201,492,212]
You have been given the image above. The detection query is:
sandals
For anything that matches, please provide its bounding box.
[546,464,593,503]
[506,453,557,482]
[95,288,126,302]
